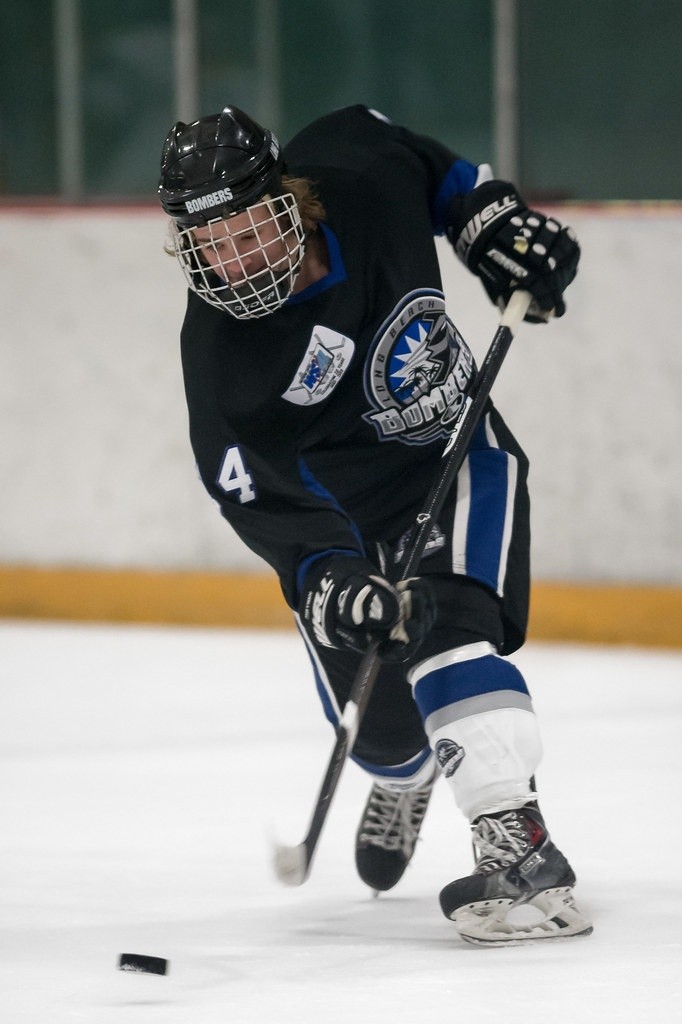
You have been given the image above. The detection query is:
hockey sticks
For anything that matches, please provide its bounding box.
[265,284,533,889]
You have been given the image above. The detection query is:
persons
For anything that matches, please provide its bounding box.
[154,101,595,945]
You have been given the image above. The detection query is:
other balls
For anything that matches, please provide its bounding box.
[119,953,167,976]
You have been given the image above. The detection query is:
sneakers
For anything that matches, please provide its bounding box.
[353,765,439,895]
[436,776,595,946]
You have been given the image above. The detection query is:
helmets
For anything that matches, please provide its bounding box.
[157,104,305,322]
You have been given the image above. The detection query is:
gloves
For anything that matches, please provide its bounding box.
[298,556,439,665]
[446,179,583,327]
